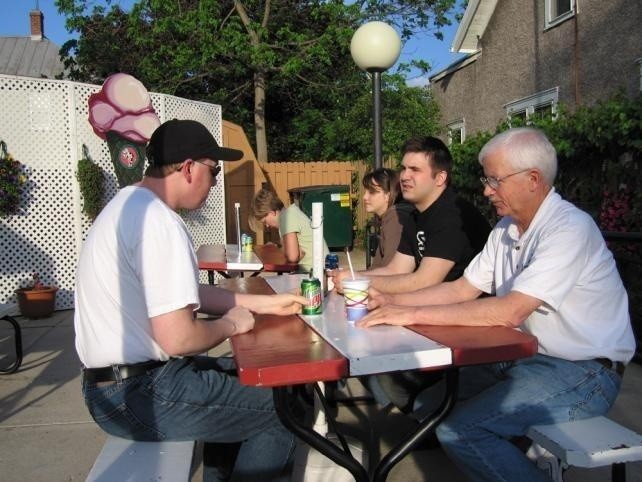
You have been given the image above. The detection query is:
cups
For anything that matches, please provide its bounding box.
[342,277,370,323]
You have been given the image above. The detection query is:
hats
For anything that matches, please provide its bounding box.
[145,119,244,164]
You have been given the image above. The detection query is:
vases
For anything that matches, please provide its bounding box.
[14,286,58,319]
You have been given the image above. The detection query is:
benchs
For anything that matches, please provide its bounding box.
[524,416,641,481]
[1,304,24,374]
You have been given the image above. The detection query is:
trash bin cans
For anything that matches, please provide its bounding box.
[287,185,353,248]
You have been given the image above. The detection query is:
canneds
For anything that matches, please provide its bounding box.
[302,279,323,316]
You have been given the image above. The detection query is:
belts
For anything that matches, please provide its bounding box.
[83,361,168,383]
[594,358,625,377]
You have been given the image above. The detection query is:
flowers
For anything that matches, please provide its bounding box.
[32,272,44,289]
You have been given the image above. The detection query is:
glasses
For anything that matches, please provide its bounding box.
[479,168,536,190]
[178,160,222,177]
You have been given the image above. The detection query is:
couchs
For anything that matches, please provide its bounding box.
[84,434,196,482]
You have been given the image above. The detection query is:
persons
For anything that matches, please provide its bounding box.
[73,119,298,482]
[345,126,637,482]
[248,188,330,274]
[326,168,417,416]
[332,135,493,449]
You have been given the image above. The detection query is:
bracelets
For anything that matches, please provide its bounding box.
[223,316,238,337]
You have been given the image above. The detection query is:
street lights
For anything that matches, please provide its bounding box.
[349,18,402,171]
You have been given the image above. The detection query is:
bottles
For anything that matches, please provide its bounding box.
[246,237,253,252]
[241,234,248,252]
